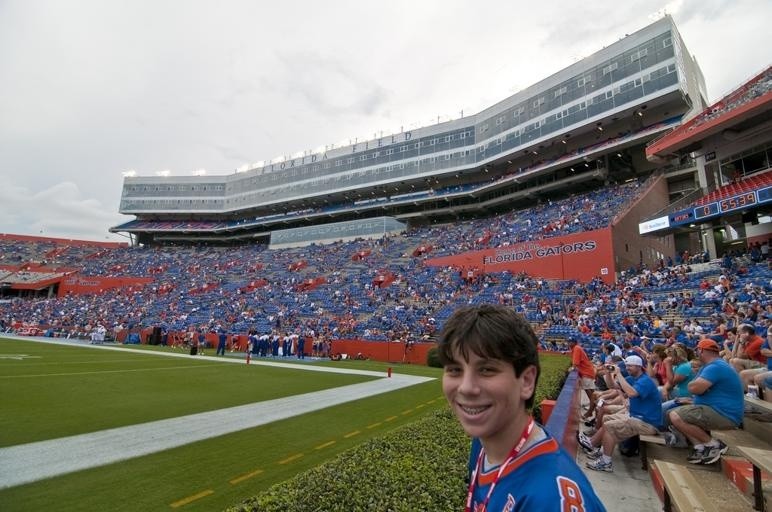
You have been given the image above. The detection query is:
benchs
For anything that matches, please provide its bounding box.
[0,177,771,363]
[610,367,771,511]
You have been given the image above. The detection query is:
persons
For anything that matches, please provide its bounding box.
[0,176,771,382]
[567,322,772,472]
[436,301,607,512]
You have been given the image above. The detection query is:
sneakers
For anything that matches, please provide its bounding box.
[687,439,729,465]
[577,431,594,453]
[586,448,613,473]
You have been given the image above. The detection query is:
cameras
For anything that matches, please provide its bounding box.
[606,365,615,371]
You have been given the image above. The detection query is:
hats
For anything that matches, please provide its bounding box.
[625,355,647,374]
[694,339,720,352]
